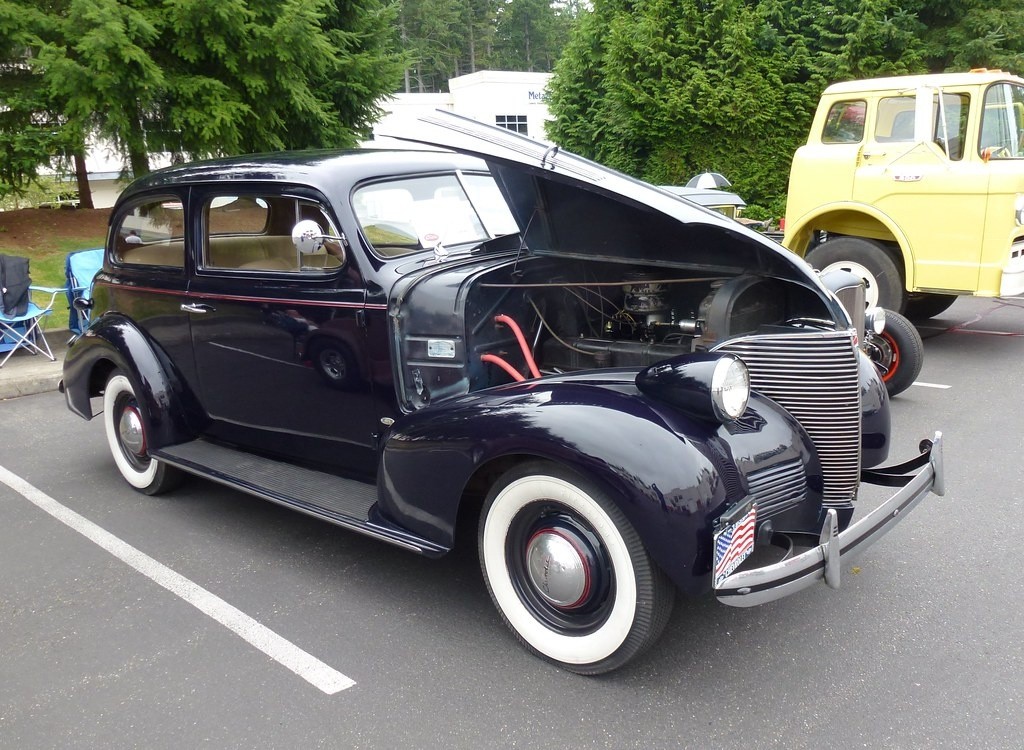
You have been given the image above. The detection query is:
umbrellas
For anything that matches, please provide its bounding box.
[685,169,732,189]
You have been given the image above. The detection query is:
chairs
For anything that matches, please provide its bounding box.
[62,246,105,335]
[0,252,70,368]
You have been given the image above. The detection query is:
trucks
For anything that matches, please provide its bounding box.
[359,67,1024,318]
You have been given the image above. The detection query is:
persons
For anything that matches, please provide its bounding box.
[125,230,142,243]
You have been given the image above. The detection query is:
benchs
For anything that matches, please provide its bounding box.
[120,235,329,270]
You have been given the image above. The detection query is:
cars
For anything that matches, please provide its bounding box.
[58,107,944,677]
